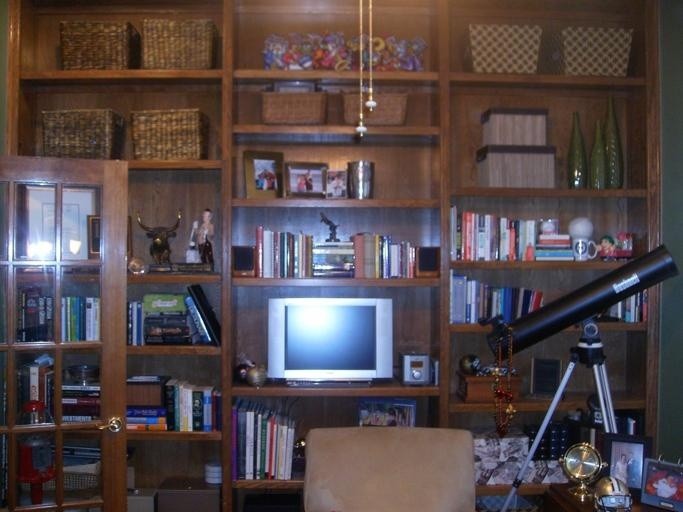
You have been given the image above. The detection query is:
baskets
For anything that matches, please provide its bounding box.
[258,90,329,126]
[129,106,205,161]
[138,17,215,70]
[57,19,131,70]
[341,91,408,126]
[39,107,125,159]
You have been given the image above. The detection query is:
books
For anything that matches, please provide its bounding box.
[448,206,575,323]
[609,290,649,324]
[253,223,416,282]
[16,285,220,433]
[232,397,298,484]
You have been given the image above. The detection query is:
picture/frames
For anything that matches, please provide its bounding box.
[607,436,647,489]
[640,457,683,511]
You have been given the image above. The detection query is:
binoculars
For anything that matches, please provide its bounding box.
[486,243,679,365]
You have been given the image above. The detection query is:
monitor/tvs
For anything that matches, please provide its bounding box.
[267,298,394,388]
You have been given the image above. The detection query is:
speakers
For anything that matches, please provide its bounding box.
[415,246,441,277]
[231,246,256,277]
[397,350,431,386]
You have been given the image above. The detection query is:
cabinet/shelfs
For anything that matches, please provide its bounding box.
[0,0,661,512]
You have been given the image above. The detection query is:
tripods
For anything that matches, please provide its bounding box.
[501,317,618,512]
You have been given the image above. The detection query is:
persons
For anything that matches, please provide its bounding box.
[305,169,313,192]
[653,470,678,499]
[332,175,342,197]
[296,174,305,191]
[262,169,275,190]
[626,450,638,484]
[255,171,265,191]
[615,452,627,484]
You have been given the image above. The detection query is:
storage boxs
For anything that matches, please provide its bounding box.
[481,107,547,143]
[126,376,166,407]
[552,24,631,77]
[474,146,556,187]
[457,23,542,74]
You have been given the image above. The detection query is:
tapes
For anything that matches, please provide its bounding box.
[204,463,222,484]
